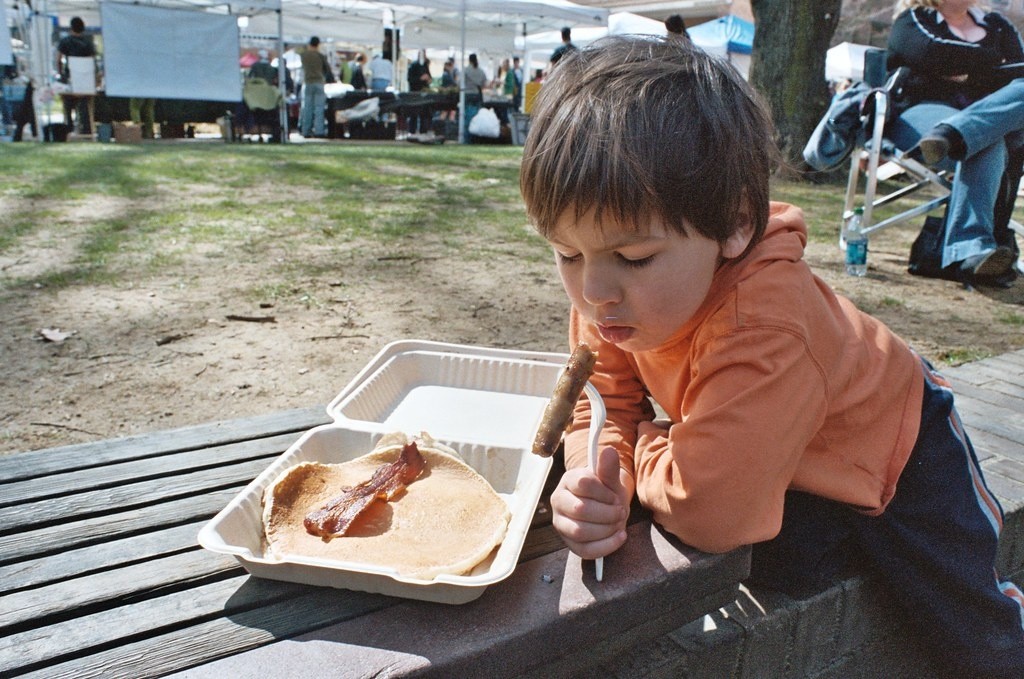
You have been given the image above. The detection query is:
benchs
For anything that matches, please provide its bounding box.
[0,362,751,679]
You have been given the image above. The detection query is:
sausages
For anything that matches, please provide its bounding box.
[531,339,600,459]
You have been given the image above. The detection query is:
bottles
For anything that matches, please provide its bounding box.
[844,207,869,279]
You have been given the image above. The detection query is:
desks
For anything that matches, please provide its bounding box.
[325,89,511,143]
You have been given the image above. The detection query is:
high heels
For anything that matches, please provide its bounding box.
[955,244,1014,294]
[902,123,959,164]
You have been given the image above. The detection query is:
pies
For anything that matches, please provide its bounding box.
[261,431,511,581]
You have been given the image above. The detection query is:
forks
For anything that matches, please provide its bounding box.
[582,380,608,581]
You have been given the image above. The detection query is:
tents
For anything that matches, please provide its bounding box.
[513,0,883,115]
[2,0,607,142]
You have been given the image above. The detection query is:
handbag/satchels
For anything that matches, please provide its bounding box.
[469,107,500,137]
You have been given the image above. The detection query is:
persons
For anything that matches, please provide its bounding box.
[517,33,1024,678]
[34,12,689,138]
[829,0,1023,279]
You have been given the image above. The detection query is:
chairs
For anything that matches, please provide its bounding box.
[839,47,1024,258]
